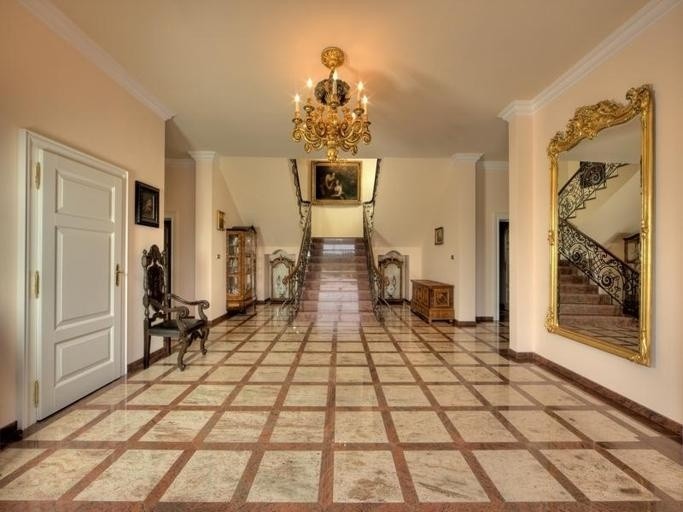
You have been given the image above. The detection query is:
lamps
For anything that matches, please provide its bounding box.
[290,46,371,164]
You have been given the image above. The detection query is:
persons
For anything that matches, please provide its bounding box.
[141,195,153,216]
[324,177,342,200]
[320,171,335,199]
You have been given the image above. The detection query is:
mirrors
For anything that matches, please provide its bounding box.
[549,82,655,372]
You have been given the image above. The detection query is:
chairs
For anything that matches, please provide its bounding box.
[140,244,210,372]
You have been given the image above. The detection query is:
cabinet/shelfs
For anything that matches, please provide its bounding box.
[410,279,455,324]
[225,225,257,314]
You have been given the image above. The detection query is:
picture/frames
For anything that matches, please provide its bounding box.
[309,160,362,206]
[134,180,160,228]
